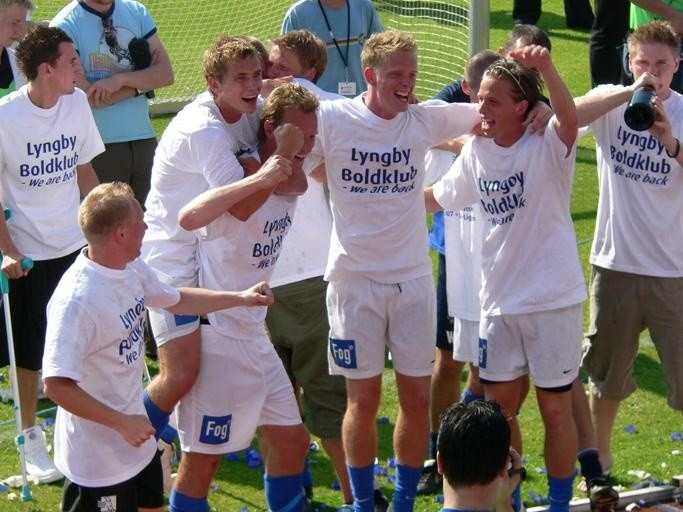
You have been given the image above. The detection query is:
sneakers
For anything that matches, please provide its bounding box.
[414,457,443,498]
[584,475,619,509]
[11,424,66,485]
[372,487,389,512]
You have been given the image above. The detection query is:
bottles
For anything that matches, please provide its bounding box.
[623,81,658,132]
